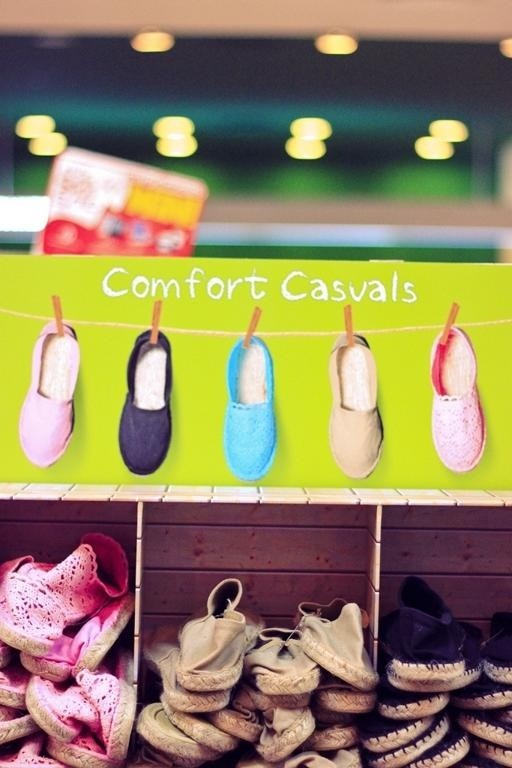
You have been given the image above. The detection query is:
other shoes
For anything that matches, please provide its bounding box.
[137,573,511,767]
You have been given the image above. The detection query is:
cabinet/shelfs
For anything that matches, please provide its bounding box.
[0,482,511,767]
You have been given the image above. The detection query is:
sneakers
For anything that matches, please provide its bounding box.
[20,322,80,468]
[329,335,384,478]
[430,328,486,473]
[0,531,138,768]
[119,329,172,474]
[224,337,275,482]
